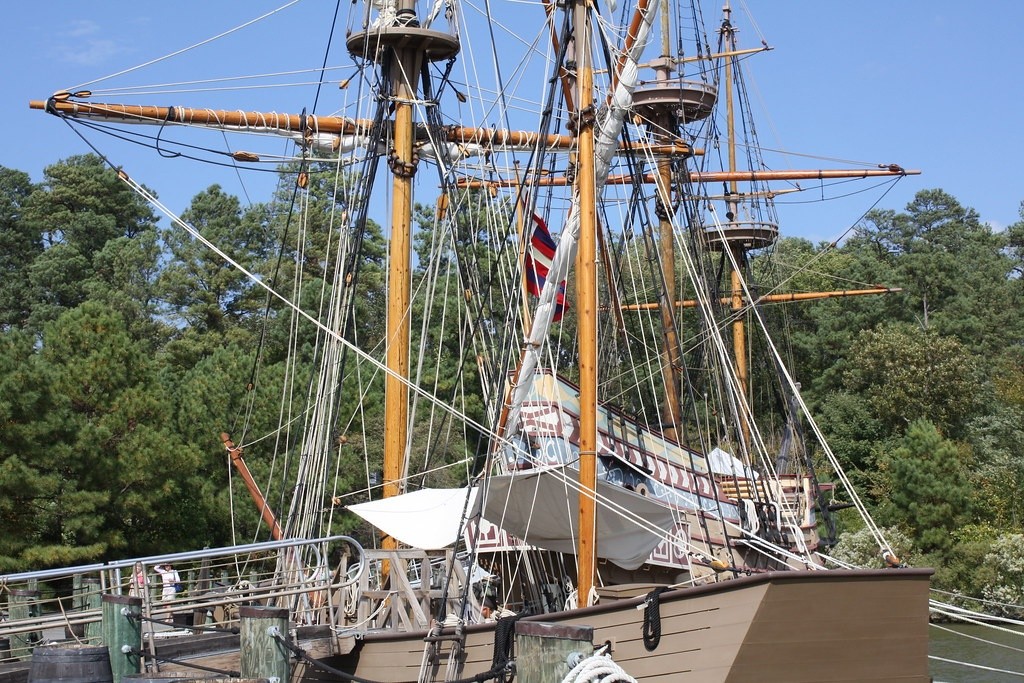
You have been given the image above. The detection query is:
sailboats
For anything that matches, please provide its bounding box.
[29,0,936,683]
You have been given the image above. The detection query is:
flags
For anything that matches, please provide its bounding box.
[521,183,570,324]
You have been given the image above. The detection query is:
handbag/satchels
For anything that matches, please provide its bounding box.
[173,570,184,592]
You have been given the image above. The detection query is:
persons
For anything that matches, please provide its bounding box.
[154,562,180,623]
[128,561,150,623]
